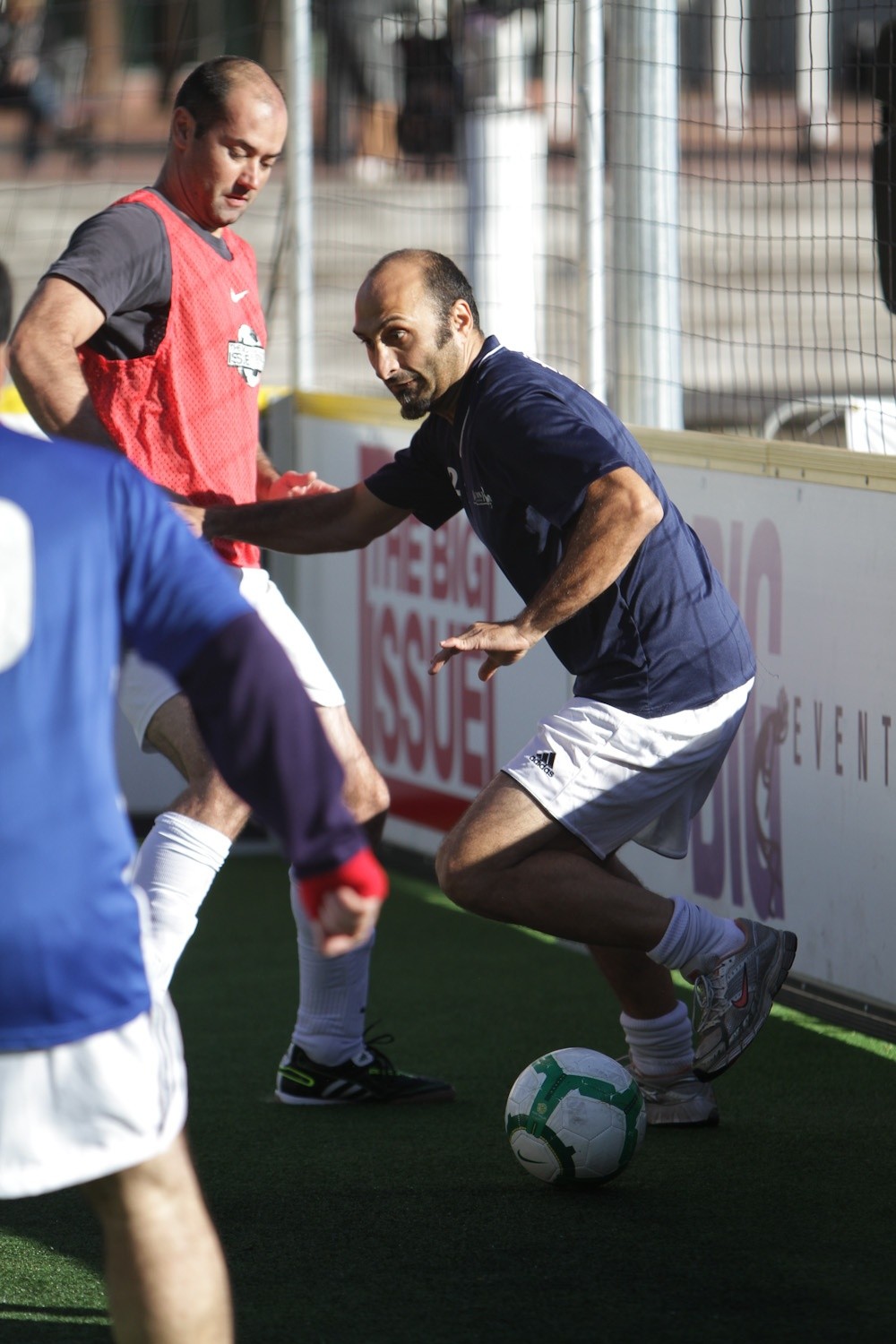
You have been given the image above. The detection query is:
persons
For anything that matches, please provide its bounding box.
[10,57,455,1109]
[349,0,459,180]
[159,248,797,1125]
[0,255,388,1344]
[6,0,96,168]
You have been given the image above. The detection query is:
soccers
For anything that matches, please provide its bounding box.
[505,1047,649,1193]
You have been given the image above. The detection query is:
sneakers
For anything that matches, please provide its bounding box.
[274,1025,456,1105]
[625,1064,721,1129]
[690,918,797,1082]
